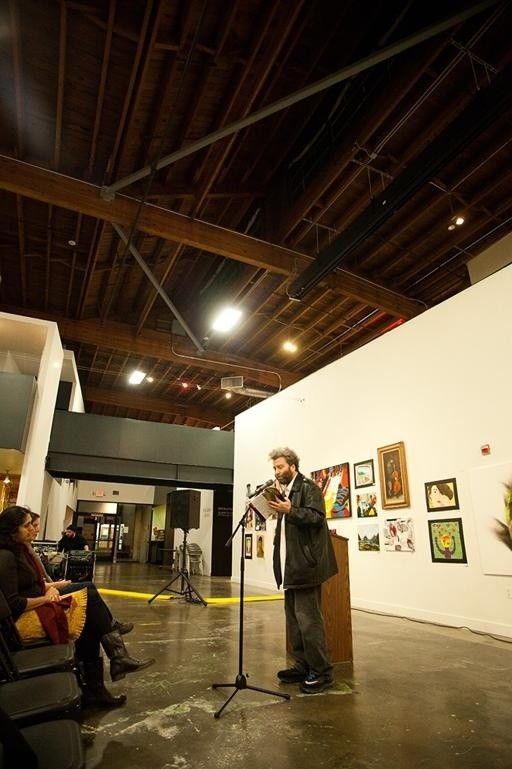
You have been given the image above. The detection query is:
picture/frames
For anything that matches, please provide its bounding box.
[353,459,375,489]
[244,533,253,560]
[425,516,468,564]
[423,477,460,514]
[375,440,411,510]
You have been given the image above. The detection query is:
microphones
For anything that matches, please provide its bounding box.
[248,479,274,499]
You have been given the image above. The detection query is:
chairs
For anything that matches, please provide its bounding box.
[0,584,88,768]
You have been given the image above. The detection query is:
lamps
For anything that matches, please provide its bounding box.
[283,48,510,304]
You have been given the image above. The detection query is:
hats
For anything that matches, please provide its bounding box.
[66,524,76,532]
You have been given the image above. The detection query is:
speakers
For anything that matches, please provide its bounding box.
[165,490,201,529]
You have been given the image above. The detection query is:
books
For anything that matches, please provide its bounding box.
[249,487,285,522]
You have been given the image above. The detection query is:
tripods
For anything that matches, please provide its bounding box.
[148,532,208,607]
[212,505,291,718]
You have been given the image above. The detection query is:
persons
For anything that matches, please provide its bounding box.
[263,449,337,694]
[0,506,156,709]
[386,459,399,498]
[358,495,376,517]
[319,469,329,490]
[429,483,454,508]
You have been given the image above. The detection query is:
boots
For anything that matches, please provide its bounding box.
[100,630,156,682]
[82,657,127,708]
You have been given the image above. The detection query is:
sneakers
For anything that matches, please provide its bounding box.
[277,663,308,683]
[113,621,134,634]
[300,671,336,694]
